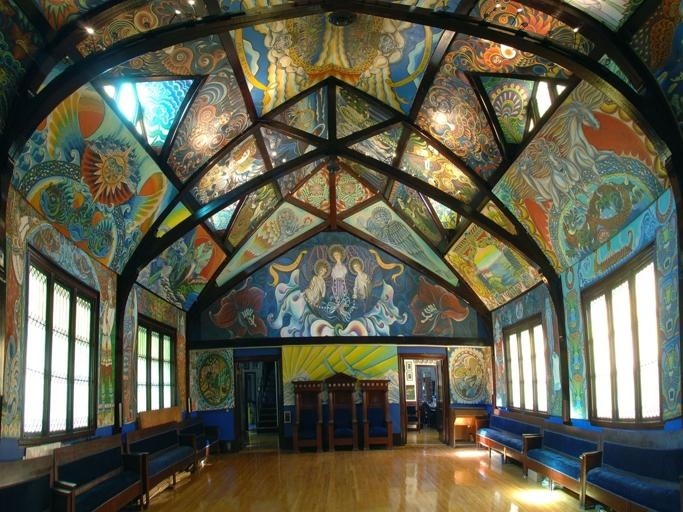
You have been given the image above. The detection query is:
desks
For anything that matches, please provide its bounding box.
[447,405,488,449]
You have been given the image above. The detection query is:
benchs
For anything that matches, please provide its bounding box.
[48,420,218,510]
[468,412,682,511]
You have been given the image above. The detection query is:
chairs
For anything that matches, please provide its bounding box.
[290,378,394,453]
[406,405,423,433]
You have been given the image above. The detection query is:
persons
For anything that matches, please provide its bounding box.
[98,275,114,425]
[306,245,369,304]
